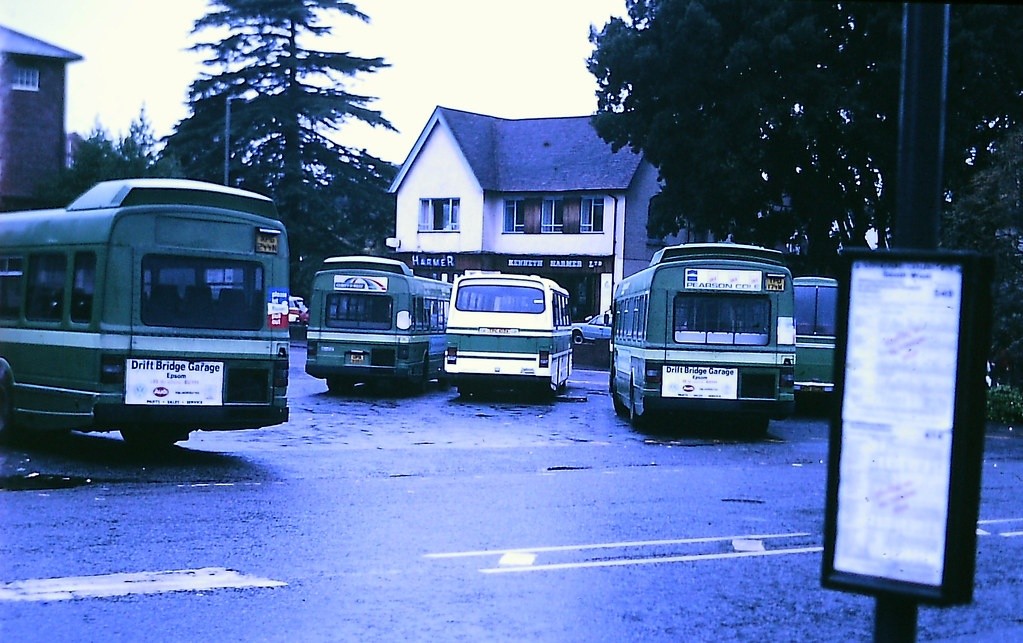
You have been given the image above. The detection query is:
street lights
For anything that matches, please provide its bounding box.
[223,90,260,187]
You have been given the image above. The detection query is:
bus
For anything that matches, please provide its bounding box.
[445,274,579,399]
[0,177,290,446]
[304,256,453,394]
[605,243,796,434]
[791,276,840,393]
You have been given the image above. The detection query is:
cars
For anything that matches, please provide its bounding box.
[287,296,308,324]
[573,314,611,343]
